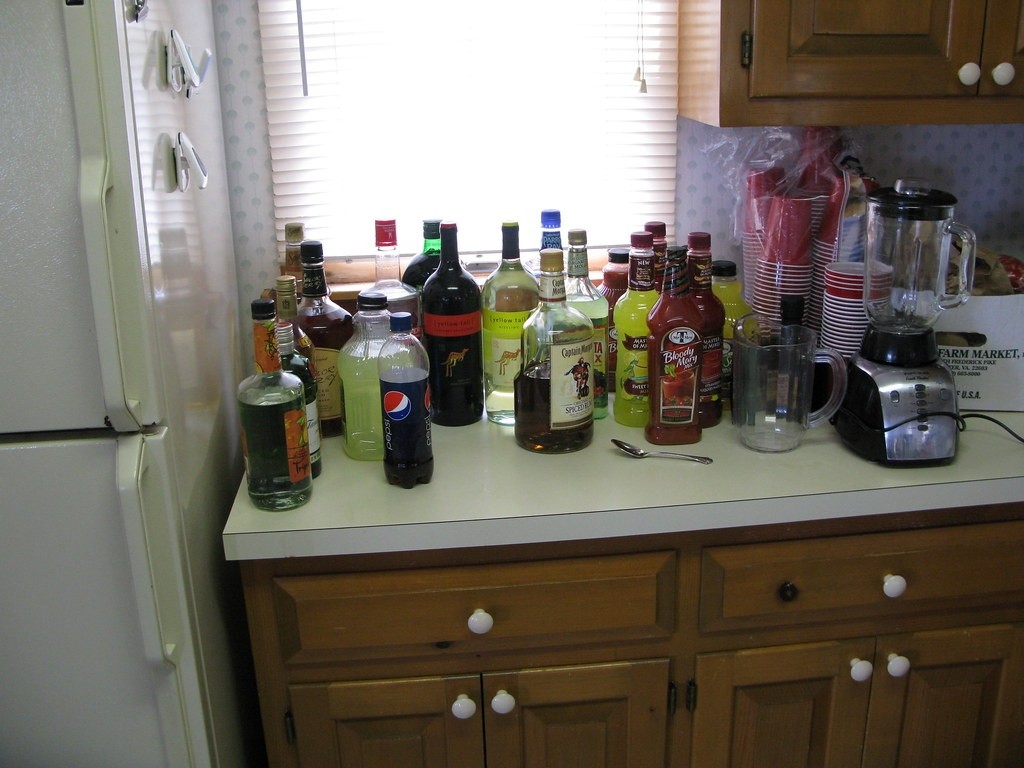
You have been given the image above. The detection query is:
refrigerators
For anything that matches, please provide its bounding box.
[0,1,269,767]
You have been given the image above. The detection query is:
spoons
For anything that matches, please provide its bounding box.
[611,439,714,465]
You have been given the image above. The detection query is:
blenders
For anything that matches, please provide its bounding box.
[834,176,976,471]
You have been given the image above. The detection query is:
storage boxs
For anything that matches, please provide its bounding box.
[892,289,1024,415]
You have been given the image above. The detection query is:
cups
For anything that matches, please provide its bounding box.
[730,312,846,452]
[740,125,893,364]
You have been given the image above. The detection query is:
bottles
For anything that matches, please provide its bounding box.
[480,221,539,426]
[378,312,434,489]
[613,232,661,428]
[686,230,726,429]
[711,260,755,411]
[644,221,667,296]
[337,291,414,461]
[421,222,484,427]
[646,246,704,445]
[563,230,609,419]
[514,249,594,455]
[357,218,423,349]
[597,248,629,393]
[524,207,569,297]
[401,218,443,345]
[236,221,353,512]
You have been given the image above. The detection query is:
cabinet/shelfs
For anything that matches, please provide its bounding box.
[243,530,688,768]
[673,518,1024,768]
[678,1,1023,128]
